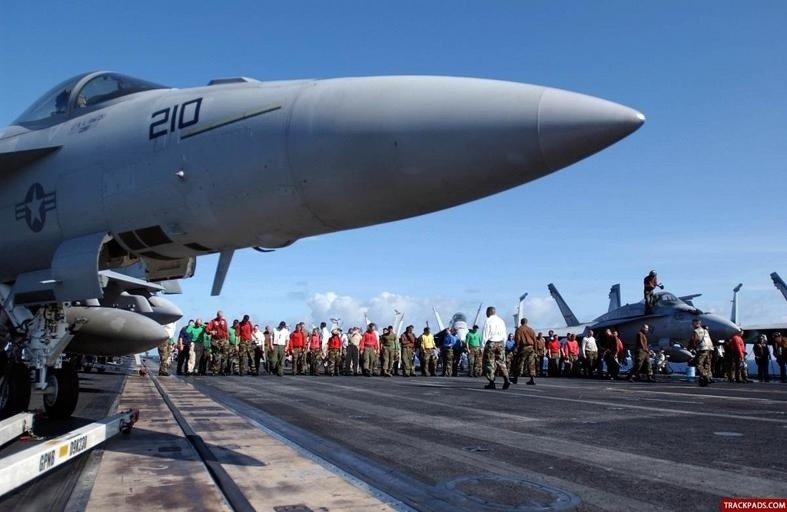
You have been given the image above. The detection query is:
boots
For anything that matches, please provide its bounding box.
[503,381,512,389]
[509,375,518,384]
[526,377,536,385]
[485,381,496,389]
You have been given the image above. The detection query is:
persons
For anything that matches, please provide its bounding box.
[154,310,787,389]
[481,306,510,389]
[643,269,656,311]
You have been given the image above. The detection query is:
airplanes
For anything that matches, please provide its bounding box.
[432,302,485,346]
[742,271,787,344]
[309,318,344,337]
[360,308,406,338]
[0,68,648,440]
[516,278,746,363]
